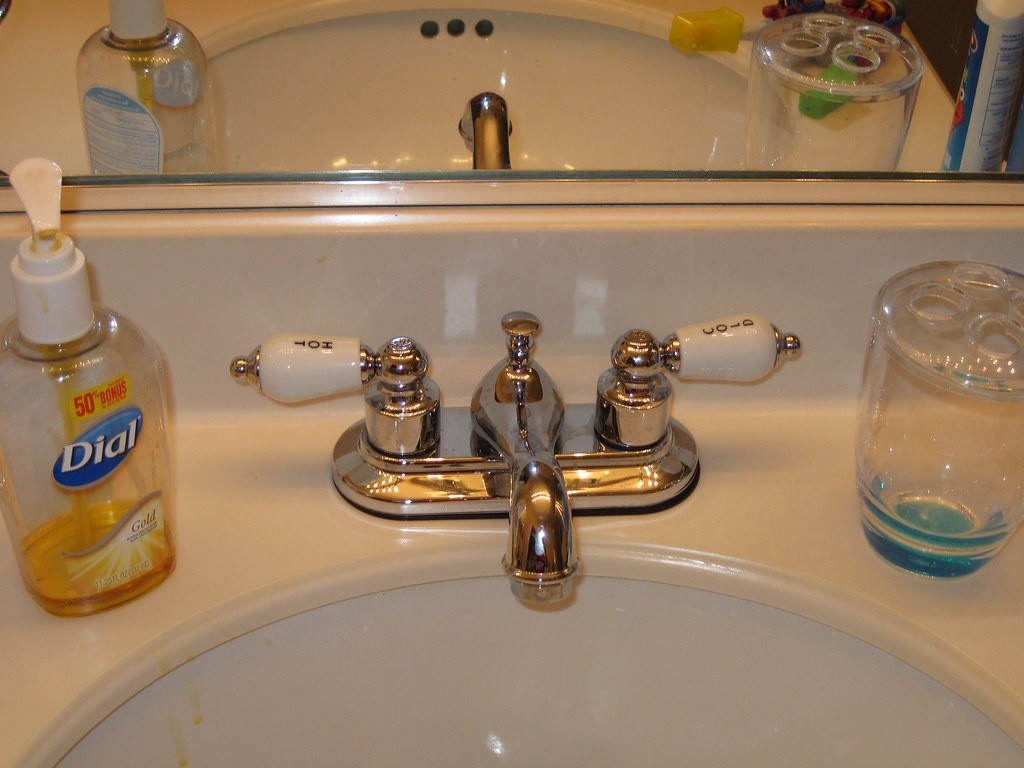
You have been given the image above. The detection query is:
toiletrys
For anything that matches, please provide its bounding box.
[76,0,224,173]
[940,0,1024,173]
[1,156,179,617]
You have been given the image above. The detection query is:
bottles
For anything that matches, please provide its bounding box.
[743,13,924,171]
[854,262,1024,578]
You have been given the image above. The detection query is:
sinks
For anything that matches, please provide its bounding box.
[71,0,800,174]
[15,533,1022,763]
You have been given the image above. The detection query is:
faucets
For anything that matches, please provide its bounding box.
[457,91,515,169]
[471,310,578,607]
